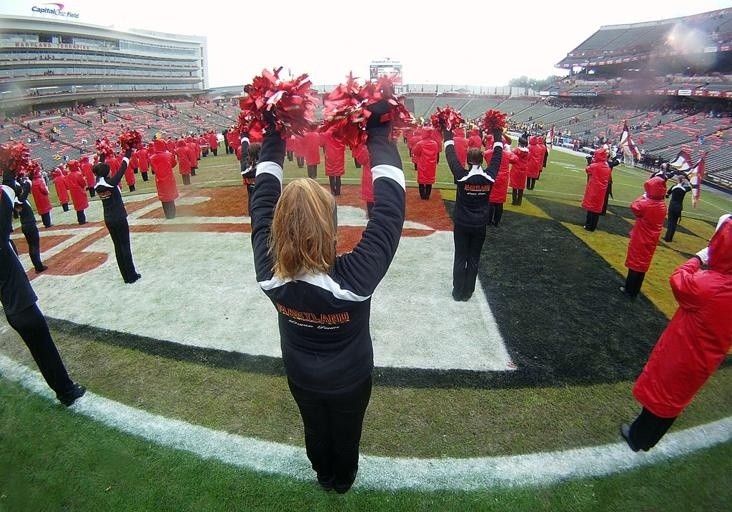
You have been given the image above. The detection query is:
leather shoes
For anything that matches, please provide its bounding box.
[620,422,639,451]
[620,287,631,297]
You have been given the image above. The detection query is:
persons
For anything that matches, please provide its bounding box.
[250,95,407,492]
[0,171,88,407]
[618,212,730,452]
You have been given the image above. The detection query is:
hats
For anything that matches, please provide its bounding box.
[92,164,110,178]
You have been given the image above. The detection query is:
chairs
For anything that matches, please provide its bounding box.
[542,6,732,97]
[402,93,570,136]
[548,105,690,160]
[621,112,732,192]
[0,97,255,190]
